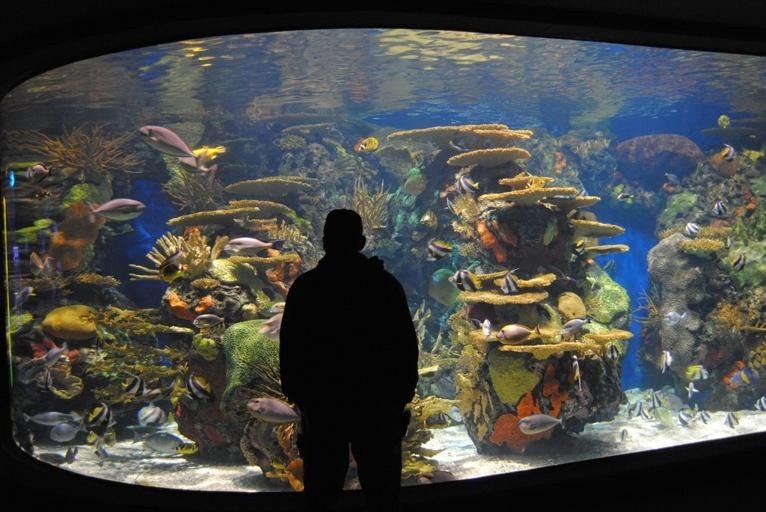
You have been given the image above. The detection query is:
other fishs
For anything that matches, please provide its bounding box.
[11,163,301,466]
[417,174,766,444]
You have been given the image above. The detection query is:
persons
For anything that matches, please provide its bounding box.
[280,209,417,512]
